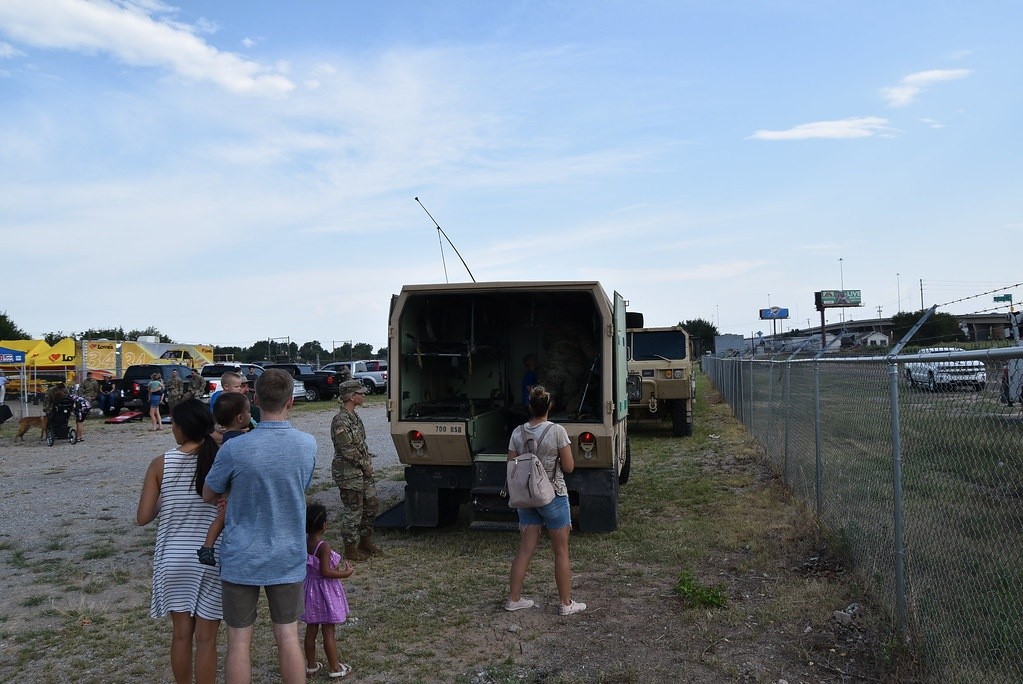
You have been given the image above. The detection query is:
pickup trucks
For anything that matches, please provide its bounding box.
[262,363,344,402]
[96,364,193,413]
[321,360,388,396]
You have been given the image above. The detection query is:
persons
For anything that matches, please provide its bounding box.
[696,357,702,372]
[233,367,242,374]
[43,383,92,442]
[521,320,599,413]
[196,369,318,684]
[166,369,206,420]
[330,381,384,560]
[137,401,224,684]
[337,365,351,404]
[98,374,115,416]
[0,372,10,405]
[504,386,587,616]
[146,373,165,431]
[246,366,258,404]
[299,501,352,676]
[82,372,99,419]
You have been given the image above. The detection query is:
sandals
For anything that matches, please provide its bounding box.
[328,663,352,679]
[305,662,326,678]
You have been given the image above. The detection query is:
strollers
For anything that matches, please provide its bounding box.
[45,399,78,446]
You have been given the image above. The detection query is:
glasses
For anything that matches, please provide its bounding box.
[191,373,194,374]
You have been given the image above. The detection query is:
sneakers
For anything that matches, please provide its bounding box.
[197,546,217,566]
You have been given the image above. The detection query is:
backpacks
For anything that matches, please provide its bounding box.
[507,424,560,509]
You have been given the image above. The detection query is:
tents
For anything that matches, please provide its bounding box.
[0,338,75,419]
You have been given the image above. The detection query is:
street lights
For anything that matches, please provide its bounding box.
[838,257,844,332]
[716,303,720,333]
[896,273,900,314]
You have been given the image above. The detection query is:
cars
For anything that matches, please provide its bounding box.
[904,348,986,393]
[198,363,267,405]
[292,378,308,400]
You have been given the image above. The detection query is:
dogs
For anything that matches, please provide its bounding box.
[15,416,48,443]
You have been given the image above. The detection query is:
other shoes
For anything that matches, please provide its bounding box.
[505,596,534,611]
[559,599,586,615]
[156,427,163,431]
[77,437,85,442]
[98,410,104,415]
[344,543,368,561]
[358,536,382,556]
[110,407,115,411]
[160,401,165,405]
[148,428,156,431]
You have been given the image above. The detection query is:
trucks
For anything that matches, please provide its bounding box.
[387,281,642,534]
[625,325,695,436]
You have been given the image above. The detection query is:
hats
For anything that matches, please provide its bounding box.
[340,380,368,395]
